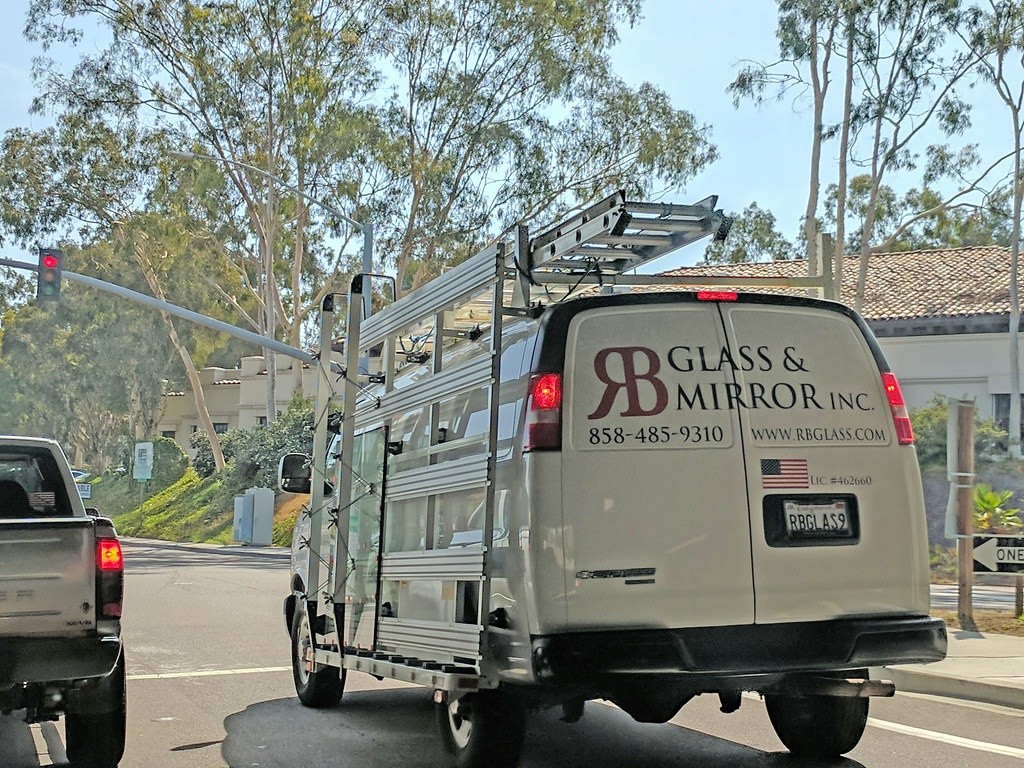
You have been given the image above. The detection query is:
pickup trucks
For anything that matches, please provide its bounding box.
[0,437,128,768]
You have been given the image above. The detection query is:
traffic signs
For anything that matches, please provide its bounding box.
[971,533,1024,575]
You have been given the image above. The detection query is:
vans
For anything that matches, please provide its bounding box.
[272,187,950,768]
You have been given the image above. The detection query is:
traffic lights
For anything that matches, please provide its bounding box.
[38,249,63,303]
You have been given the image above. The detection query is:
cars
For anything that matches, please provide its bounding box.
[70,468,92,479]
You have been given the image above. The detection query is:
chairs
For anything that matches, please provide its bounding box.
[0,479,44,519]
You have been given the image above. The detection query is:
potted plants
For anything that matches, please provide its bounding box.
[973,486,1024,573]
